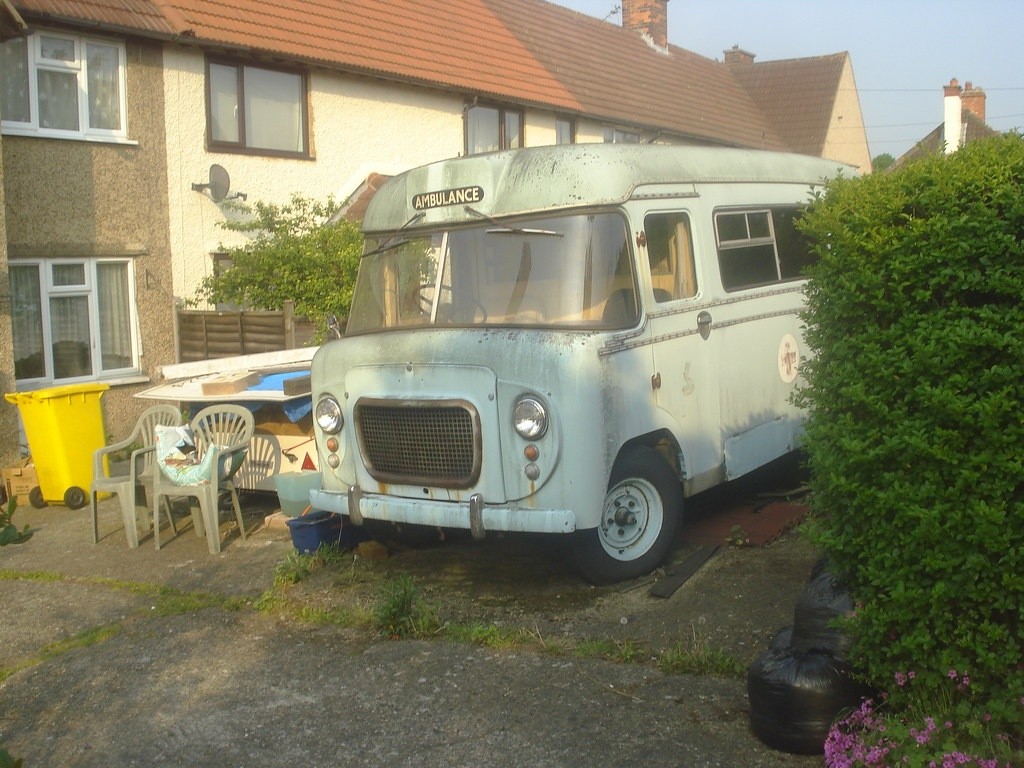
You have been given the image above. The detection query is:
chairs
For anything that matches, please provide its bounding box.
[89,405,186,548]
[149,404,255,555]
[600,287,673,328]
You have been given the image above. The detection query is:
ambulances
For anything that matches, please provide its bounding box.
[308,142,861,580]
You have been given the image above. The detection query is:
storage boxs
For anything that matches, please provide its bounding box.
[284,504,351,554]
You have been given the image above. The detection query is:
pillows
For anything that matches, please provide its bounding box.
[153,424,247,487]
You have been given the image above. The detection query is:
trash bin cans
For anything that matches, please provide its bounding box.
[4,382,112,510]
